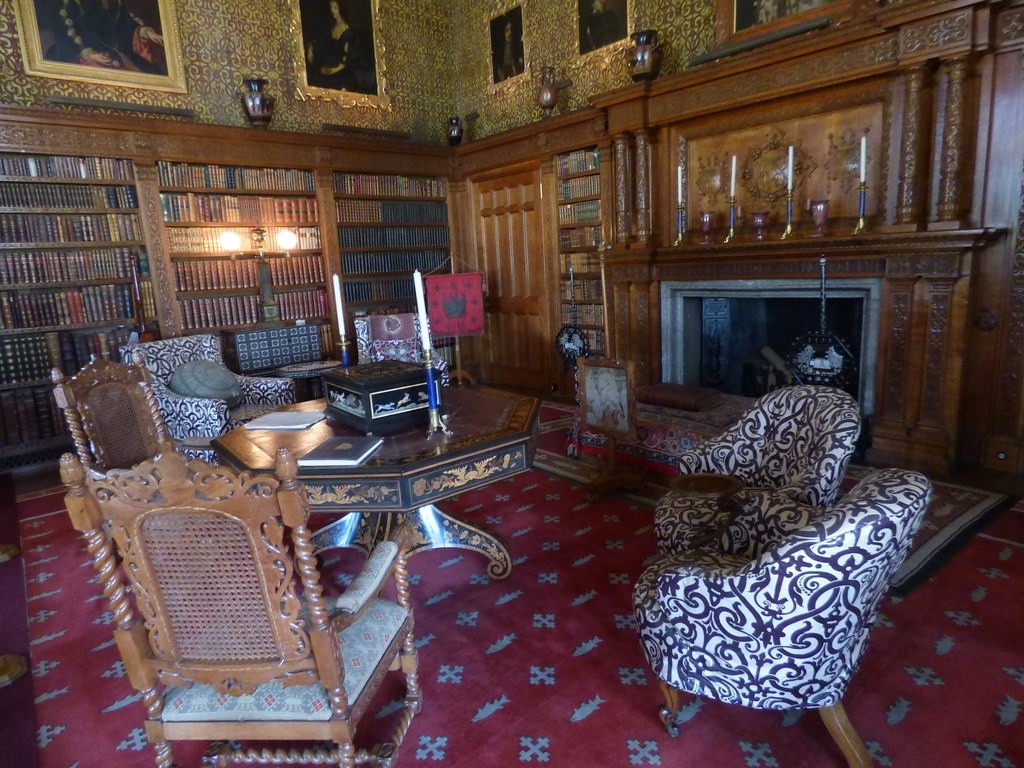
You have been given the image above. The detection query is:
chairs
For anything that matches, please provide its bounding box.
[54,444,428,768]
[48,349,219,483]
[631,466,944,768]
[117,333,302,467]
[650,384,864,559]
[354,311,452,389]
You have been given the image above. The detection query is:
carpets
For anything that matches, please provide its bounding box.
[525,405,1016,597]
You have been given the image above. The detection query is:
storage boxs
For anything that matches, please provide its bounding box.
[319,357,444,438]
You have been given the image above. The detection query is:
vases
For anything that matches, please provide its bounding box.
[752,209,772,242]
[446,116,464,143]
[625,26,666,80]
[537,65,561,117]
[240,77,280,127]
[809,198,833,237]
[700,209,716,244]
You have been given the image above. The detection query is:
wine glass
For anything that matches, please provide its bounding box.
[810,200,830,237]
[750,211,770,241]
[698,210,715,244]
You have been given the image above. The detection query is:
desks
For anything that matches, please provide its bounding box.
[213,380,545,672]
[272,359,345,400]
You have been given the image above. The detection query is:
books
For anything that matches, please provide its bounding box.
[0,157,156,448]
[320,324,332,352]
[158,161,333,329]
[433,337,452,366]
[332,172,449,303]
[297,436,384,466]
[557,150,606,350]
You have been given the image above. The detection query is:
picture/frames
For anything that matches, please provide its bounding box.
[287,0,394,114]
[708,0,862,50]
[483,0,535,96]
[576,355,639,446]
[11,0,191,96]
[569,0,639,73]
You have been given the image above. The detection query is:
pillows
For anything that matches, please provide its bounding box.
[170,358,245,411]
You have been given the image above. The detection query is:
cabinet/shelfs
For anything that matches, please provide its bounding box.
[152,156,332,358]
[335,171,457,372]
[0,150,165,460]
[554,147,606,359]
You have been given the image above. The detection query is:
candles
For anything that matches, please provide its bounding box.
[413,264,434,352]
[859,134,867,183]
[787,144,795,191]
[677,165,684,203]
[729,154,738,198]
[332,269,348,339]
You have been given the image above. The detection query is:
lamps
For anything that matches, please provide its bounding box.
[216,226,299,322]
